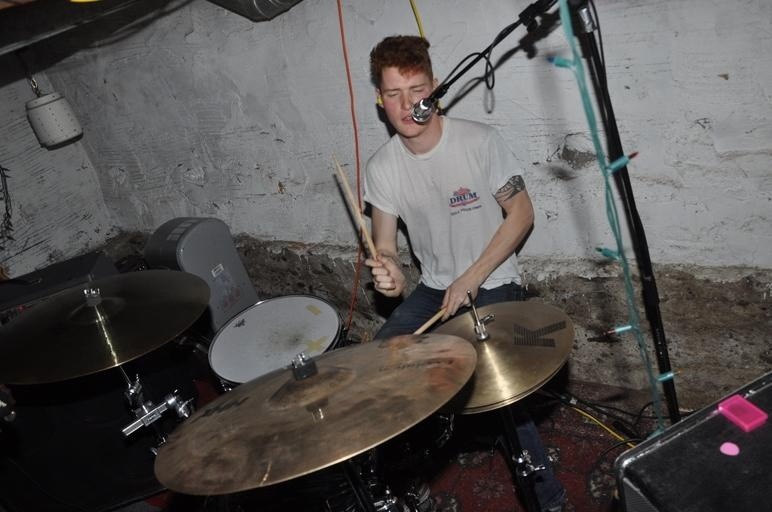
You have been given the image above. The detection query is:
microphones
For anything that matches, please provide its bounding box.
[411,97,433,124]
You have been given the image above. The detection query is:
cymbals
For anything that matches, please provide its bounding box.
[1,270,211,385]
[431,302,575,415]
[154,333,477,495]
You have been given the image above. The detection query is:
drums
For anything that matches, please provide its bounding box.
[208,295,344,393]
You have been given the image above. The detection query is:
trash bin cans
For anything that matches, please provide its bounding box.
[142,217,260,338]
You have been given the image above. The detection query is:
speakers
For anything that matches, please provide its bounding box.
[614,369,772,512]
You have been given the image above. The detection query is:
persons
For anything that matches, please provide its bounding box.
[361,34,569,510]
